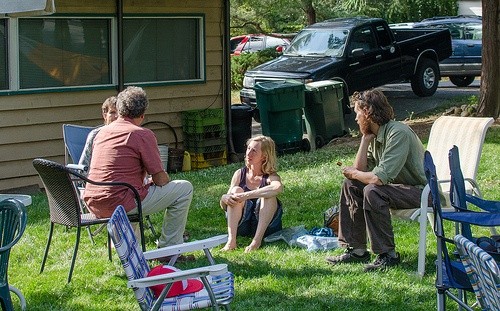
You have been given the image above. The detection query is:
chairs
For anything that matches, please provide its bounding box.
[62,123,156,243]
[0,198,27,311]
[33,158,146,282]
[107,205,235,311]
[390,114,500,311]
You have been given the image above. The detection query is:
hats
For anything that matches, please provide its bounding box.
[147,265,204,299]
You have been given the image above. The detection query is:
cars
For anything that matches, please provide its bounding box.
[229,34,298,56]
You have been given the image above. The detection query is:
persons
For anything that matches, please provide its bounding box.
[220,134,283,252]
[78,96,141,247]
[325,88,434,271]
[83,86,195,262]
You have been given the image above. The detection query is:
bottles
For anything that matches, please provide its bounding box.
[336,162,354,172]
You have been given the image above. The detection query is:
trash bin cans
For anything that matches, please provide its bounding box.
[231,102,255,154]
[253,79,311,155]
[304,79,349,152]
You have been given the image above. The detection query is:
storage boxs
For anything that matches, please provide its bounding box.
[180,108,226,169]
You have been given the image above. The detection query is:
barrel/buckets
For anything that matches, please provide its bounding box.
[157,146,169,171]
[177,150,192,171]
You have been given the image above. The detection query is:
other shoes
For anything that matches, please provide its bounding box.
[363,252,401,273]
[326,244,371,265]
[160,254,195,264]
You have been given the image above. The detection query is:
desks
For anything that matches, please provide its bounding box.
[0,194,33,311]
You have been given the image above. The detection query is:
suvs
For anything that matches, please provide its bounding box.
[388,14,483,86]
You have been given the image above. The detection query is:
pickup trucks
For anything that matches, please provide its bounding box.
[239,16,453,124]
